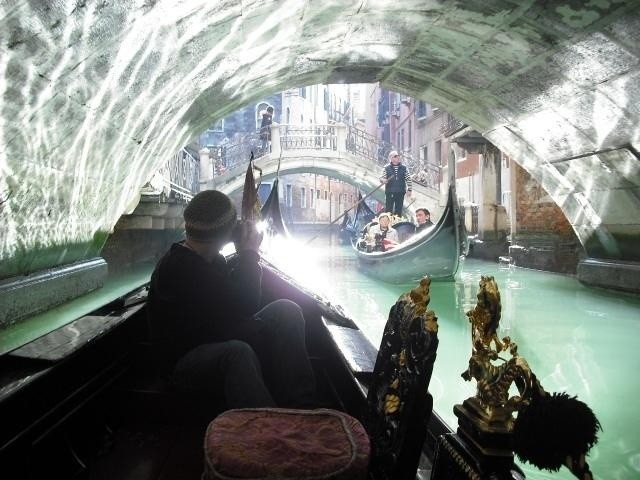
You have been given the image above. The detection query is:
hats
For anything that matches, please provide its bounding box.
[183,190,236,241]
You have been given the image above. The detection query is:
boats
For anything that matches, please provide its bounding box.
[352,182,466,284]
[337,188,377,244]
[241,147,292,248]
[0,251,521,480]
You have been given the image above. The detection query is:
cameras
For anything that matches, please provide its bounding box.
[231,219,253,241]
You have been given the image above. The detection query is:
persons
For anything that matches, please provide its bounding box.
[366,212,400,252]
[414,208,436,235]
[258,104,274,158]
[378,150,413,218]
[146,190,320,412]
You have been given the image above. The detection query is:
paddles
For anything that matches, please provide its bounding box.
[306,175,395,243]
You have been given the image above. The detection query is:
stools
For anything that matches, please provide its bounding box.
[202,407,372,480]
